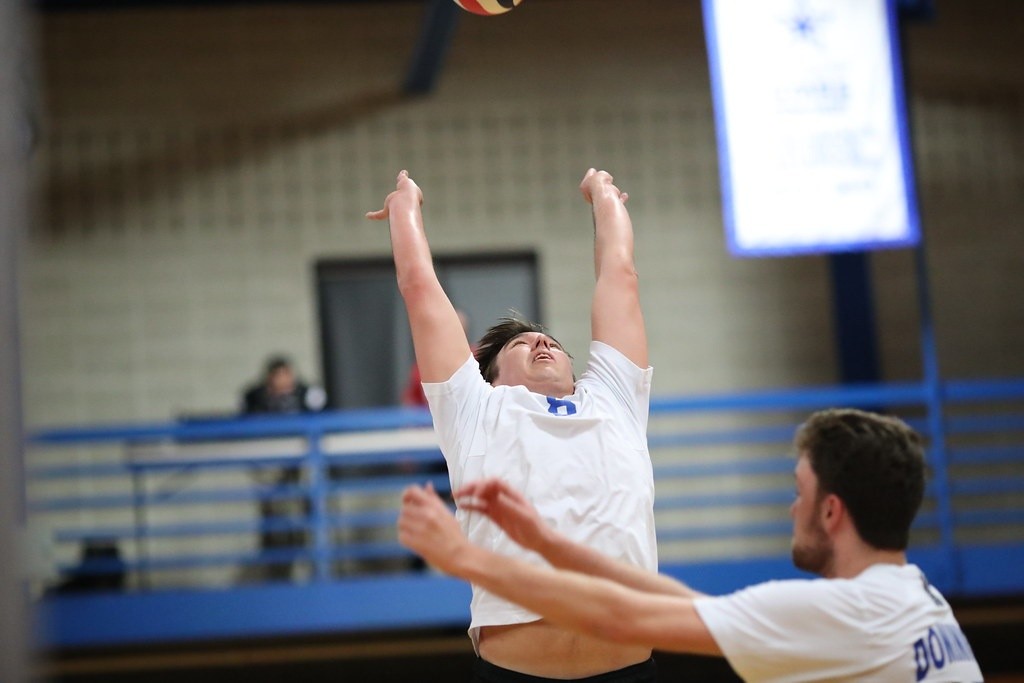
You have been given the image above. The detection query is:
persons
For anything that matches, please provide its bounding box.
[396,412,986,682]
[405,313,481,572]
[238,355,333,578]
[363,166,662,683]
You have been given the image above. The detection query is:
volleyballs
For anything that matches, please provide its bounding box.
[449,0,523,15]
